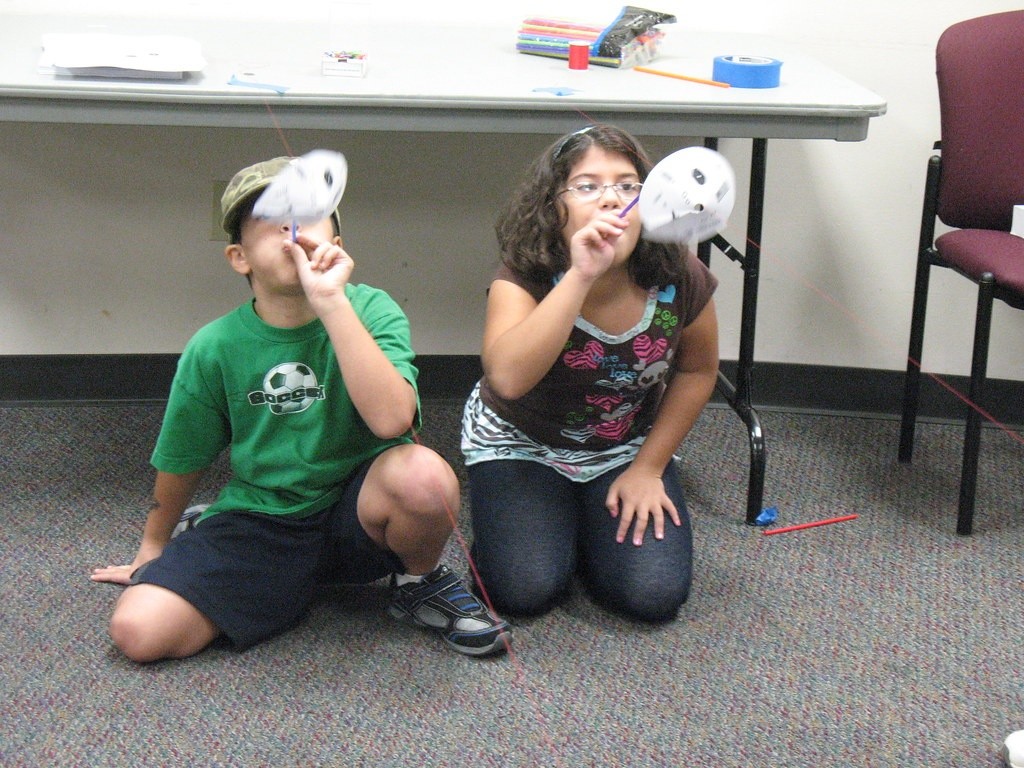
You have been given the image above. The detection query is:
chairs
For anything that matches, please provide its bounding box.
[897,9,1024,535]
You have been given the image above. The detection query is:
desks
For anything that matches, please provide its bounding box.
[0,62,888,526]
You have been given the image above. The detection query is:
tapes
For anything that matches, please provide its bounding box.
[713,55,784,89]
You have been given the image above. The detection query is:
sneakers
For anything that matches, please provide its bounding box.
[388,564,512,655]
[170,503,216,540]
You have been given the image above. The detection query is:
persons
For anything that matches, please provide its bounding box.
[92,155,514,663]
[462,123,720,622]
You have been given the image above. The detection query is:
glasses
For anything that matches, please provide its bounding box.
[551,183,643,203]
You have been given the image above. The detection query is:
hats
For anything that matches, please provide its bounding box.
[220,157,342,244]
